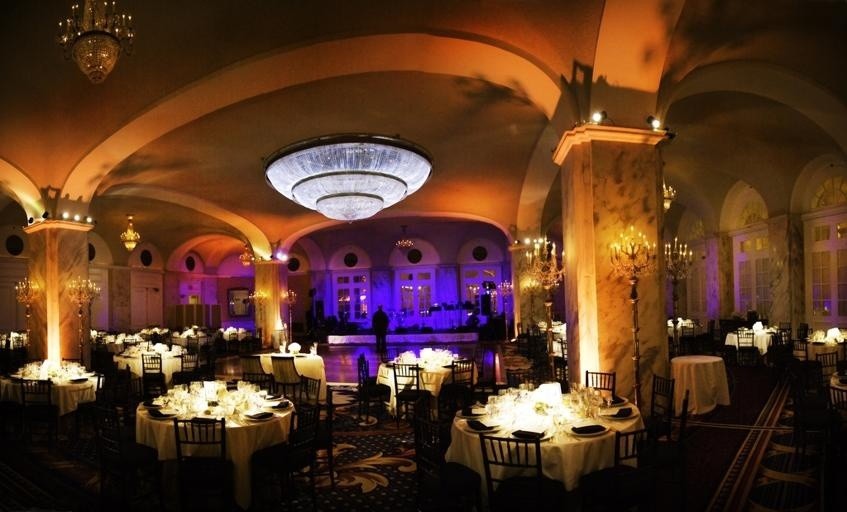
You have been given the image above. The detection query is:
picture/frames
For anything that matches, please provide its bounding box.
[226,286,252,318]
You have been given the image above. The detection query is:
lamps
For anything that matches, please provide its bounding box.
[66,274,101,367]
[663,236,692,357]
[282,288,298,344]
[392,225,415,250]
[249,290,269,350]
[238,243,254,267]
[662,178,676,213]
[56,0,138,86]
[120,214,141,253]
[14,276,42,365]
[497,279,512,345]
[608,224,655,420]
[592,110,607,124]
[646,114,661,129]
[263,132,435,226]
[524,237,565,383]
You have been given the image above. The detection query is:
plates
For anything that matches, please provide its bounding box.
[142,380,291,425]
[456,382,639,443]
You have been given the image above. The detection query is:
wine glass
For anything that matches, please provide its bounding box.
[387,347,464,370]
[1,359,91,384]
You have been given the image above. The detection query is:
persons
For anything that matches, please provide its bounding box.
[372,306,389,352]
